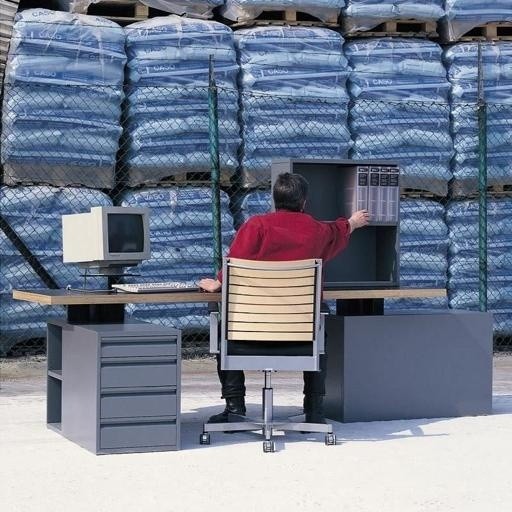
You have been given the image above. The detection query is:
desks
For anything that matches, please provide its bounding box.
[12,286,447,325]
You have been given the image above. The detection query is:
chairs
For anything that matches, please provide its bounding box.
[199,258,337,452]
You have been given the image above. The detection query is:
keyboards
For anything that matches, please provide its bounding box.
[111,280,216,294]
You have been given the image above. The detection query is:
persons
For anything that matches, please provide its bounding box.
[197,173,371,434]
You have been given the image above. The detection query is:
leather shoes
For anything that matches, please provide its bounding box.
[300,394,327,434]
[207,397,246,434]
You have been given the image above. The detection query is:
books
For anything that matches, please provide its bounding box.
[339,165,398,223]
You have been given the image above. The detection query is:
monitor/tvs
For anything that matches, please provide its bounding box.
[62,206,150,276]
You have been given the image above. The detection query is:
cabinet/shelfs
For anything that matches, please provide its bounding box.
[46,316,182,456]
[271,159,400,289]
[317,310,493,423]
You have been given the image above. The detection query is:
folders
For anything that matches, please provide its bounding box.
[343,166,369,218]
[368,166,380,223]
[388,167,400,223]
[379,166,389,223]
